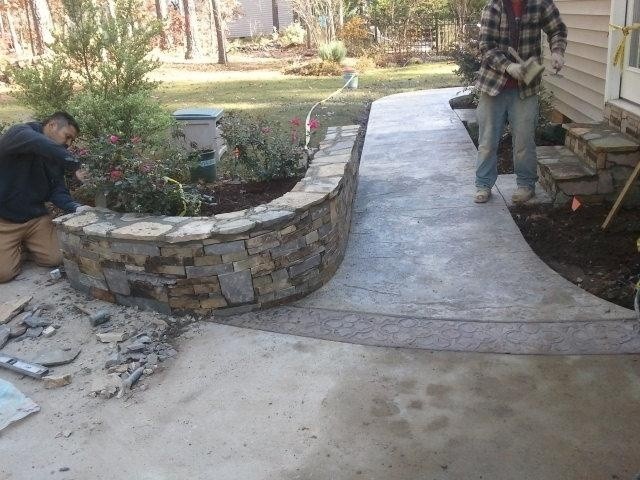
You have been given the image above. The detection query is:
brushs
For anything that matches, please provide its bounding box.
[508,45,546,86]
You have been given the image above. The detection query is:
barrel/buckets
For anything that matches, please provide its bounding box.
[344,71,358,88]
[188,149,217,183]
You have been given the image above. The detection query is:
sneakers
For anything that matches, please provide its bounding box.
[473,186,491,203]
[511,184,535,202]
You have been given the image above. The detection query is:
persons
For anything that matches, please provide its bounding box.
[471,0,569,207]
[0,110,91,285]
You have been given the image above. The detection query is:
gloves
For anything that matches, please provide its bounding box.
[549,52,566,73]
[505,62,527,81]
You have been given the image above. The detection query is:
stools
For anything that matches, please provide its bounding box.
[172,107,227,162]
